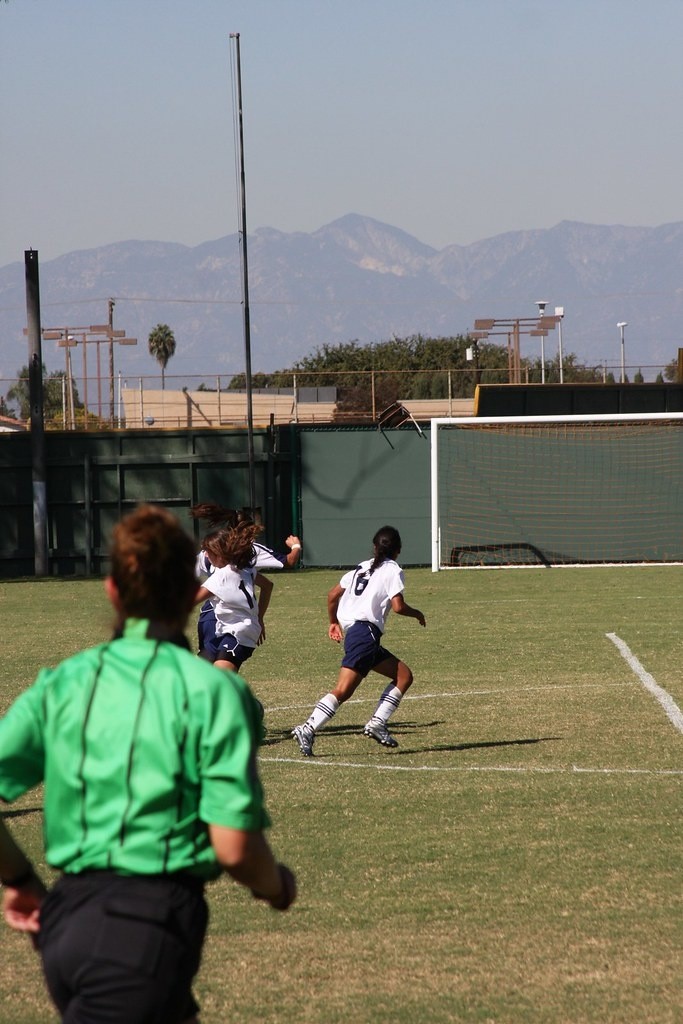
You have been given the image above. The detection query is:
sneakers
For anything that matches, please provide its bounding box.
[363,720,399,748]
[290,726,314,758]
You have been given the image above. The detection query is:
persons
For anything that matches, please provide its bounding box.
[190,504,301,664]
[0,504,295,1024]
[290,526,426,755]
[193,520,273,673]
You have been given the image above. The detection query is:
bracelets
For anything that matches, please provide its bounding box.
[291,544,301,550]
